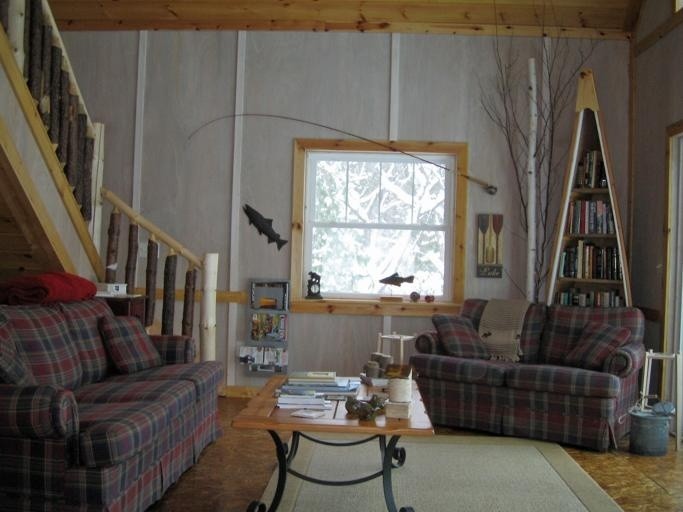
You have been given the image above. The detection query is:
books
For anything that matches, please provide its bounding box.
[555,149,626,308]
[252,313,286,341]
[277,372,361,420]
[238,346,288,368]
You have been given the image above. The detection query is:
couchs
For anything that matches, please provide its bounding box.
[2,300,223,511]
[407,299,643,451]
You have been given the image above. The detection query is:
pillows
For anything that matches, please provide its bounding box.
[102,313,162,371]
[1,336,36,389]
[431,315,492,361]
[562,321,632,369]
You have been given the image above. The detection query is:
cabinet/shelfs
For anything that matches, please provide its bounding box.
[548,66,632,310]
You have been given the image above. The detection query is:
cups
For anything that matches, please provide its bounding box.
[367,351,390,380]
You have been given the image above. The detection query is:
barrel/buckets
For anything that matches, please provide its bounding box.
[627,395,674,456]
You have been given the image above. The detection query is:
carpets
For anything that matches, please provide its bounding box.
[256,430,624,512]
